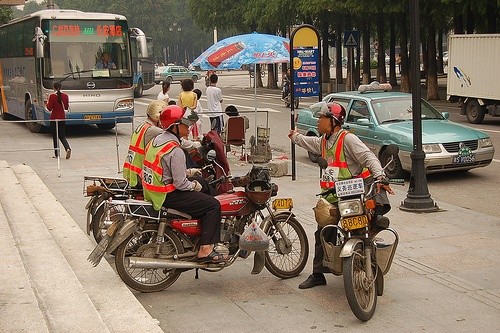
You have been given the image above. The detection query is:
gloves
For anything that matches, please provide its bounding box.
[192,180,202,192]
[188,168,203,178]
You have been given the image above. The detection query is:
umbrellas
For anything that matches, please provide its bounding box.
[191,31,291,162]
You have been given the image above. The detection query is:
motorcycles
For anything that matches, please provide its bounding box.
[248,65,265,78]
[82,129,309,293]
[315,157,400,321]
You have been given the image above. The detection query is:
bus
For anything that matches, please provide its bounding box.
[129,33,157,97]
[0,9,149,133]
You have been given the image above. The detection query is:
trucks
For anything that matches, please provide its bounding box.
[445,32,500,124]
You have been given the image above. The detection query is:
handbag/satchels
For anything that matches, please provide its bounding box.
[239,221,270,252]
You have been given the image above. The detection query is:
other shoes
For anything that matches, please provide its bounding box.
[299,274,326,289]
[66,149,71,159]
[370,214,390,232]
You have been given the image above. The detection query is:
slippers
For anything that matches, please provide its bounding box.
[197,249,228,264]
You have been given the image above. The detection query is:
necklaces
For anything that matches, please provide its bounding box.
[209,85,215,87]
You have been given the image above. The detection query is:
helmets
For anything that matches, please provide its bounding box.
[245,180,273,205]
[145,100,169,122]
[309,101,346,125]
[159,105,199,131]
[312,197,340,227]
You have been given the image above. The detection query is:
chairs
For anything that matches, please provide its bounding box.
[223,116,248,163]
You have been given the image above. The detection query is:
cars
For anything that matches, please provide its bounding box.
[295,79,495,178]
[342,57,348,68]
[373,52,390,65]
[443,51,448,65]
[154,62,202,85]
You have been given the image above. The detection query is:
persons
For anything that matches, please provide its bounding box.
[46,82,71,159]
[123,99,167,194]
[206,73,224,135]
[94,52,116,69]
[142,105,229,263]
[157,79,206,148]
[288,101,394,289]
[220,106,249,152]
